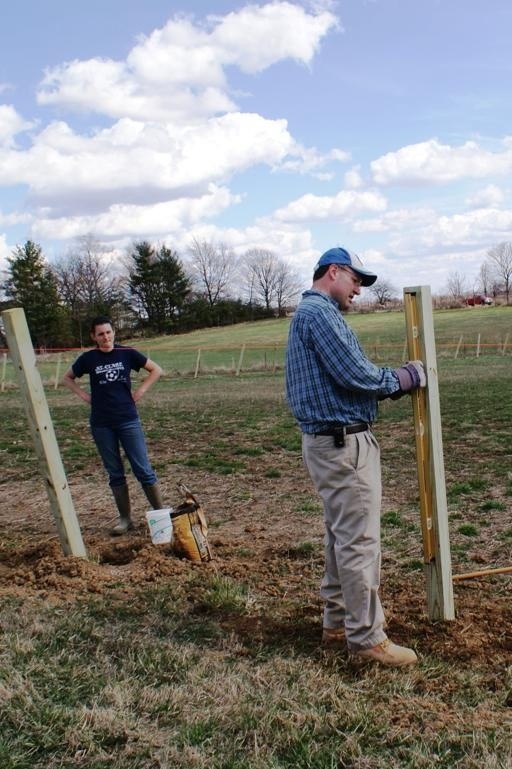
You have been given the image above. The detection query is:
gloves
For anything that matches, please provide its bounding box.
[389,359,427,400]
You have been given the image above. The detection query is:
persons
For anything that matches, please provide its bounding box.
[284,247,428,668]
[60,317,163,536]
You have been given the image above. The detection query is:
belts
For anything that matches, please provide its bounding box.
[307,423,368,438]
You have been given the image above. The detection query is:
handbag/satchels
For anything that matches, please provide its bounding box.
[169,491,213,563]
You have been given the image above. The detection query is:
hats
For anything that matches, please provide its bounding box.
[313,246,378,287]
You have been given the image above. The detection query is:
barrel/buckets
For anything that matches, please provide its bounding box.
[146,507,175,545]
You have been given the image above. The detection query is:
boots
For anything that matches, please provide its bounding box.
[109,482,133,536]
[141,481,164,511]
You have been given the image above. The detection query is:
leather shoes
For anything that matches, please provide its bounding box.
[321,626,349,648]
[348,638,417,668]
